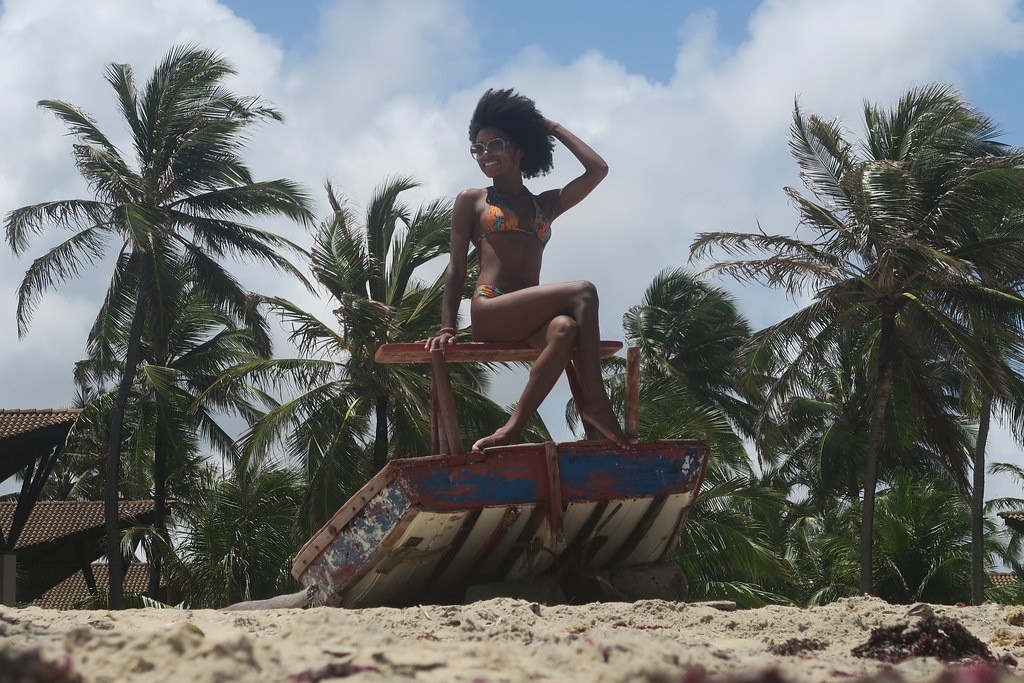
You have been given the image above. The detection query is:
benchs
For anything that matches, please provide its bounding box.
[374,334,622,454]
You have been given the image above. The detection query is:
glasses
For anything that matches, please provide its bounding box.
[470,138,515,160]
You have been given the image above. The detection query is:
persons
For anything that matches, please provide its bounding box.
[414,87,638,453]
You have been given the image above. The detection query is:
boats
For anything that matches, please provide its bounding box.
[290,332,708,610]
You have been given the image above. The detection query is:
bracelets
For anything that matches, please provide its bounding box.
[439,327,456,332]
